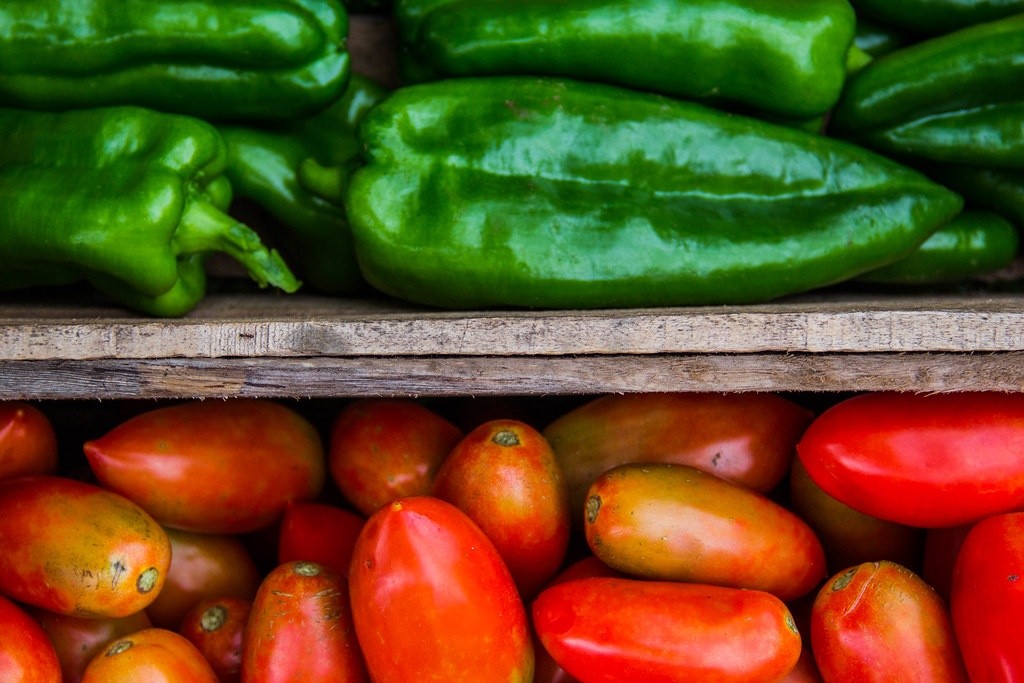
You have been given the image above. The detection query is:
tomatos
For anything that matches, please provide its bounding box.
[1,393,1024,683]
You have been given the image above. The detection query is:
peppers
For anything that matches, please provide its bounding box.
[0,0,1024,308]
